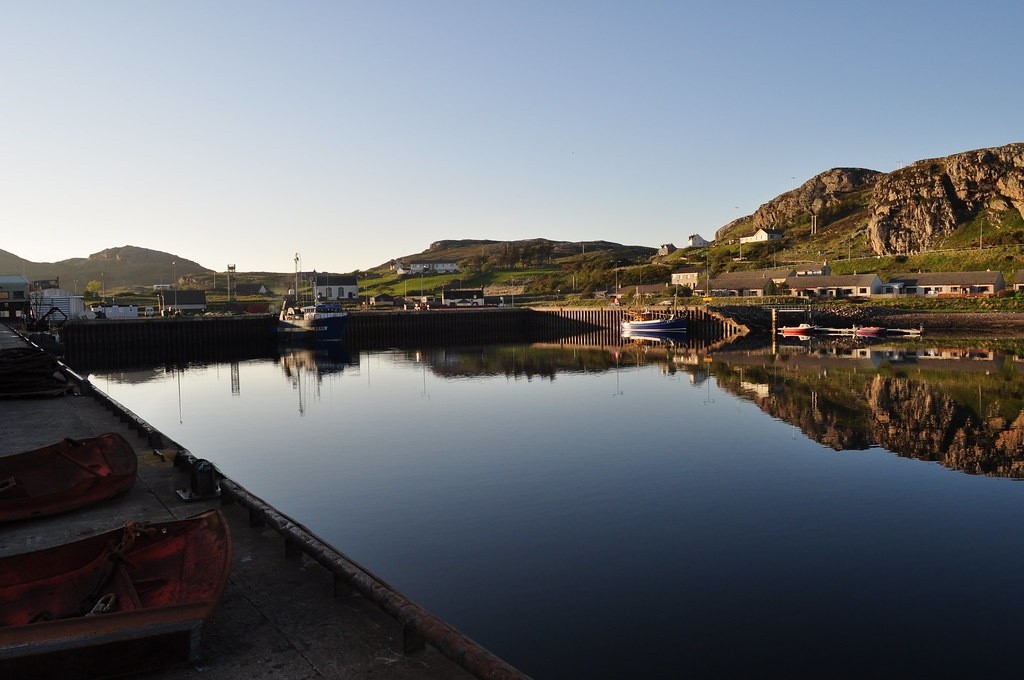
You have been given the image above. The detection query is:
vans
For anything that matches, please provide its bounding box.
[145,307,154,317]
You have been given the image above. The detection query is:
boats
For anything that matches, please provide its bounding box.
[852,326,888,337]
[0,432,138,518]
[0,510,233,662]
[277,301,349,344]
[777,323,815,336]
[0,347,52,392]
[621,317,687,335]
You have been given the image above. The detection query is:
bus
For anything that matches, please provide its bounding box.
[442,288,485,308]
